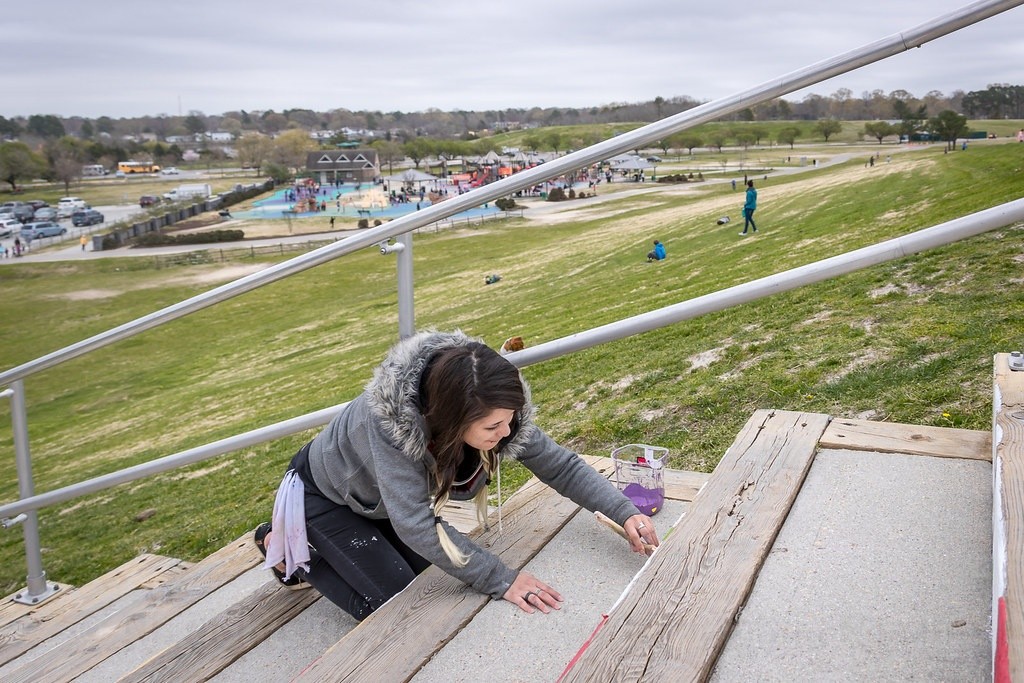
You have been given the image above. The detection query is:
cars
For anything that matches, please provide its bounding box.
[647,155,662,163]
[252,161,261,168]
[116,170,126,178]
[0,200,50,240]
[161,166,179,175]
[241,162,250,169]
[33,206,59,222]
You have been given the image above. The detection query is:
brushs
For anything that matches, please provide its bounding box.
[591,511,656,556]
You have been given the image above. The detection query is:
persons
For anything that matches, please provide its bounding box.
[253,327,660,613]
[80,235,87,250]
[1018,128,1024,142]
[0,236,20,258]
[962,142,967,150]
[217,157,892,264]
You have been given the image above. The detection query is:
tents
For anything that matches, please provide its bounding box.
[386,148,659,193]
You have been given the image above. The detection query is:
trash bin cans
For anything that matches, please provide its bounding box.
[384,185,388,191]
[454,180,459,186]
[540,192,547,198]
[448,171,452,175]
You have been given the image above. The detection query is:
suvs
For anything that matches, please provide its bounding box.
[20,220,67,241]
[58,197,91,211]
[72,208,105,227]
[139,195,161,208]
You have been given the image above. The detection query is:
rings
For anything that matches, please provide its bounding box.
[536,589,542,596]
[524,591,533,602]
[637,523,645,531]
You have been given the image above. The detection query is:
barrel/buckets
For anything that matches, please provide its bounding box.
[611,444,669,515]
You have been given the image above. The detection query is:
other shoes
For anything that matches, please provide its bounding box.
[738,232,747,235]
[752,230,759,233]
[253,522,312,590]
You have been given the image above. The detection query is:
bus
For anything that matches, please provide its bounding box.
[81,164,106,176]
[117,161,161,174]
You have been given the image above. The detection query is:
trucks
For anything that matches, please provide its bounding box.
[161,182,212,206]
